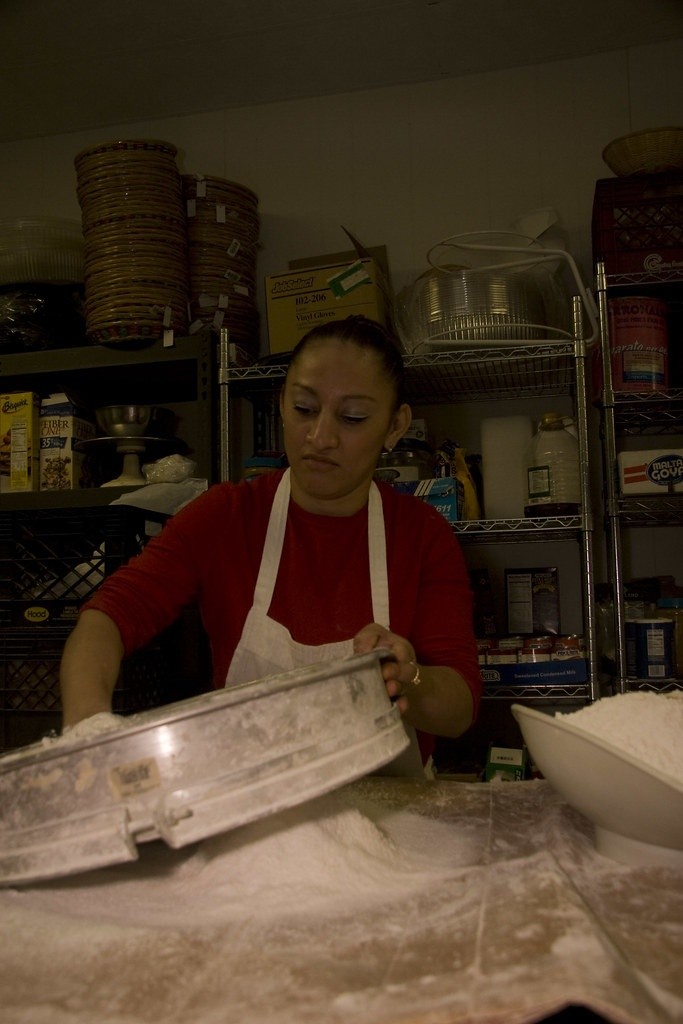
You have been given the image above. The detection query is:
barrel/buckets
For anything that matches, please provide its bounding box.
[624,619,676,679]
[608,295,670,396]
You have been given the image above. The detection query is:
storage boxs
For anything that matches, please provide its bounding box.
[0,505,175,637]
[0,638,180,752]
[264,225,394,362]
[592,172,683,284]
[617,449,683,497]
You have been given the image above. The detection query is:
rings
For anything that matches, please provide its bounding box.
[409,661,424,685]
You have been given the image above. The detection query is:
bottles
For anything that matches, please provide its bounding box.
[665,599,682,673]
[456,448,483,521]
[522,412,582,517]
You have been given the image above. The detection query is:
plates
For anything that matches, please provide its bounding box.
[73,139,259,343]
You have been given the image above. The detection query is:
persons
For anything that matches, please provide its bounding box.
[59,316,482,781]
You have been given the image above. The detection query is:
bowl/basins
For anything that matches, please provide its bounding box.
[0,216,82,284]
[95,405,156,436]
[396,271,546,366]
[510,703,683,855]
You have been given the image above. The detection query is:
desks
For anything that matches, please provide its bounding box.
[0,780,683,1024]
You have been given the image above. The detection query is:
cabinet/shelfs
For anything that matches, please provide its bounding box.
[596,260,683,693]
[0,331,240,757]
[216,295,624,773]
